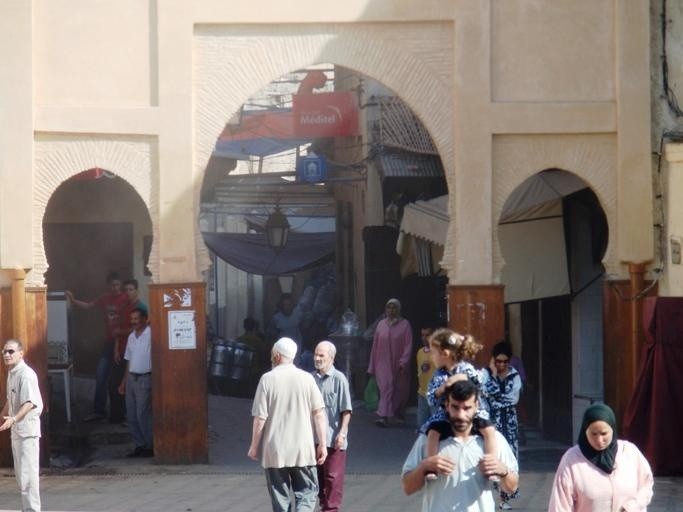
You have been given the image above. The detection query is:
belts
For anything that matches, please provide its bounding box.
[129,372,152,378]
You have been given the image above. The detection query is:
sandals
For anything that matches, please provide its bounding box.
[484,474,501,483]
[426,471,438,482]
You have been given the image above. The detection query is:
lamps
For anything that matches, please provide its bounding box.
[265,193,292,255]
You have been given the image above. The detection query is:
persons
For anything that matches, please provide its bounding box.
[236,316,267,395]
[400,379,518,512]
[262,294,303,368]
[547,402,654,511]
[482,341,524,510]
[415,326,438,432]
[425,326,501,483]
[0,337,45,511]
[117,308,153,457]
[367,298,414,425]
[246,335,329,512]
[310,340,353,511]
[111,279,150,423]
[66,269,127,423]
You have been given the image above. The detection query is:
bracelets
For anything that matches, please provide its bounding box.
[501,466,511,477]
[12,417,17,424]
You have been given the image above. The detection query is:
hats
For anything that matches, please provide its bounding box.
[274,337,298,360]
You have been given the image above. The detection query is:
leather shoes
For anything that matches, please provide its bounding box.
[138,448,153,458]
[126,448,144,458]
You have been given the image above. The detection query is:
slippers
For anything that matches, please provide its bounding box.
[394,417,404,428]
[375,418,388,427]
[498,501,511,510]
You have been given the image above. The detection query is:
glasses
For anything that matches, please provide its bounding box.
[494,360,510,365]
[2,349,21,354]
